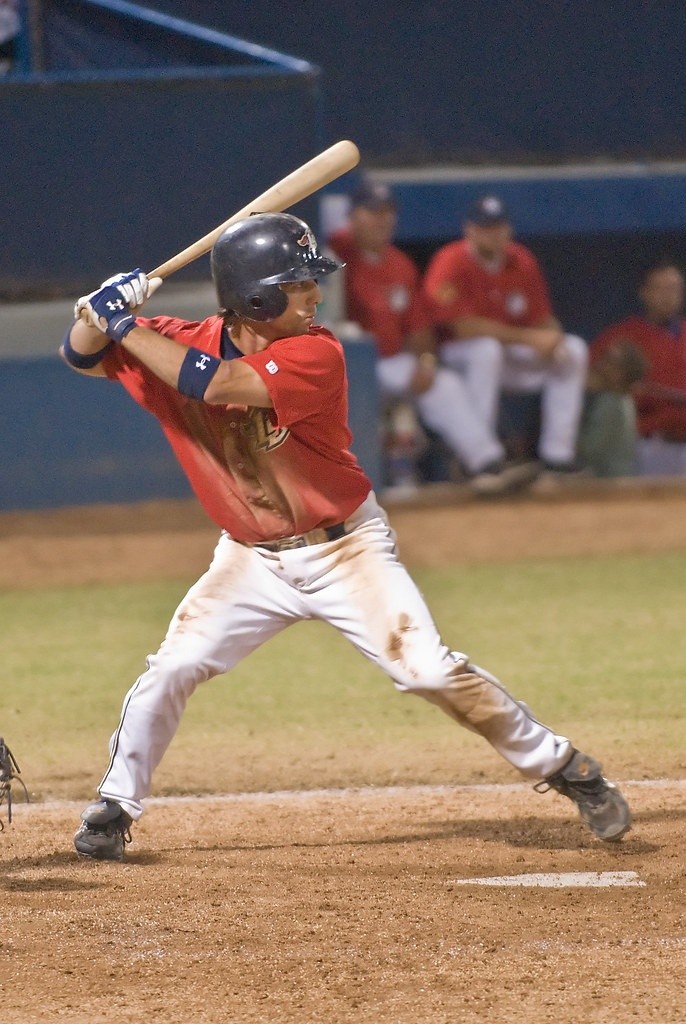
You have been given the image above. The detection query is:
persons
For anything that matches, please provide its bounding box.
[582,259,686,479]
[423,195,587,486]
[59,211,633,863]
[327,180,540,500]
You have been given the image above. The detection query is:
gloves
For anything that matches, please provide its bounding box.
[102,267,163,312]
[73,285,137,344]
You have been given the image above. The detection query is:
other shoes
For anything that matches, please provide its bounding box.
[474,453,537,489]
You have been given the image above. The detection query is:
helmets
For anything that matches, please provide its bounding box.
[212,212,343,323]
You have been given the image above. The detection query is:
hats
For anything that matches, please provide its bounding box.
[469,196,505,227]
[355,183,389,210]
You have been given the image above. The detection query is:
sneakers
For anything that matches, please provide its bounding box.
[534,749,631,841]
[73,800,132,859]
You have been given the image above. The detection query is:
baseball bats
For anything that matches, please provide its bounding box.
[80,138,360,328]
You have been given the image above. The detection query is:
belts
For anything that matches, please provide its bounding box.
[256,522,345,552]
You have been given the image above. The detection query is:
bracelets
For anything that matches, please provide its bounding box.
[63,322,113,370]
[419,352,435,361]
